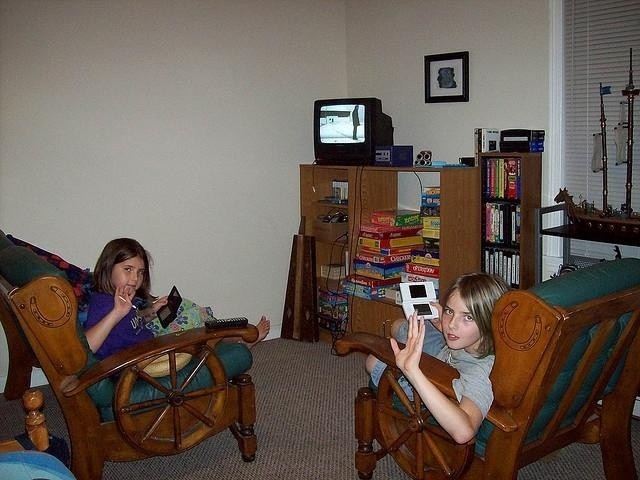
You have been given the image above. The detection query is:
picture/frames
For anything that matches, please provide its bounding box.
[423,50,469,104]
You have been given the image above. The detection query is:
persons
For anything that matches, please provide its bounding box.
[364,272,508,444]
[352,105,361,140]
[81,237,272,379]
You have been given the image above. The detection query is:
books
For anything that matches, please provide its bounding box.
[321,264,346,281]
[331,180,348,205]
[481,156,523,289]
[319,287,347,332]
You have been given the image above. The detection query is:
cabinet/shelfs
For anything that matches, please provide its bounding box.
[348,166,479,339]
[475,153,541,292]
[300,164,398,344]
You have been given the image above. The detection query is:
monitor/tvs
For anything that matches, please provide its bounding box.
[313,98,394,166]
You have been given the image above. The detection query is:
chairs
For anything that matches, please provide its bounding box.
[0,229,260,478]
[332,257,640,478]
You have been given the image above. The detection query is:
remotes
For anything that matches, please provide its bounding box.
[206,317,248,329]
